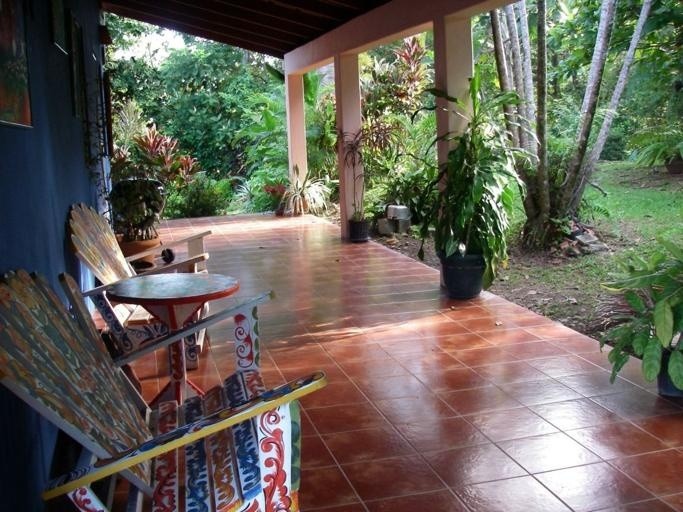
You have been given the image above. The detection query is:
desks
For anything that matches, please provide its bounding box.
[102,269,241,408]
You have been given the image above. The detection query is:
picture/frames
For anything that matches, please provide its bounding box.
[0,0,35,130]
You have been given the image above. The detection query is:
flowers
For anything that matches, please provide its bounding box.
[111,124,198,237]
[264,179,287,201]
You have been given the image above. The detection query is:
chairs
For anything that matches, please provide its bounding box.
[67,201,212,370]
[1,266,326,512]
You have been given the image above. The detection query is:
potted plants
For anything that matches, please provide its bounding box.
[596,229,683,402]
[633,123,683,174]
[338,121,402,243]
[401,64,528,301]
[285,165,330,215]
